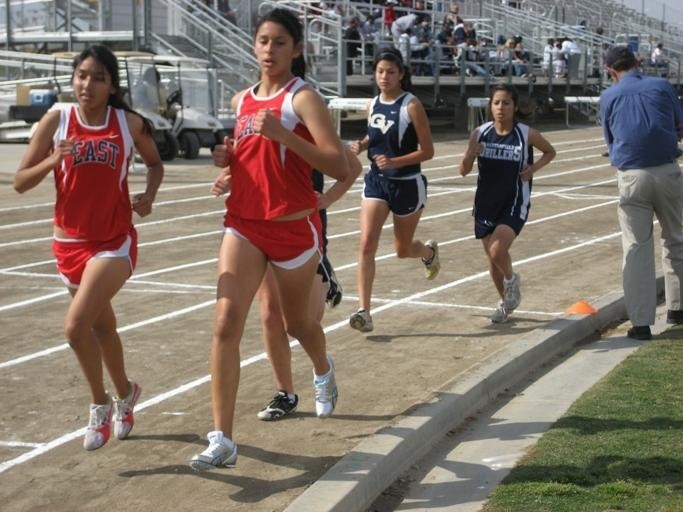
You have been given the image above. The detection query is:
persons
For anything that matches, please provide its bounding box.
[315,0,672,82]
[349,49,441,331]
[598,43,683,341]
[188,9,351,475]
[14,45,166,452]
[135,58,183,129]
[456,84,560,324]
[206,48,346,424]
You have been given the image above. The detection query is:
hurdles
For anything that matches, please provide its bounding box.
[467,97,491,135]
[326,99,372,143]
[564,95,600,128]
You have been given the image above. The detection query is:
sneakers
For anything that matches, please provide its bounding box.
[313,353,338,418]
[113,381,142,440]
[257,391,298,420]
[325,274,343,308]
[421,240,440,280]
[492,301,513,324]
[82,391,114,450]
[628,326,652,340]
[350,308,373,333]
[190,431,237,471]
[666,310,683,325]
[504,273,521,309]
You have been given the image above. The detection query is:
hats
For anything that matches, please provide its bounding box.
[607,46,634,79]
